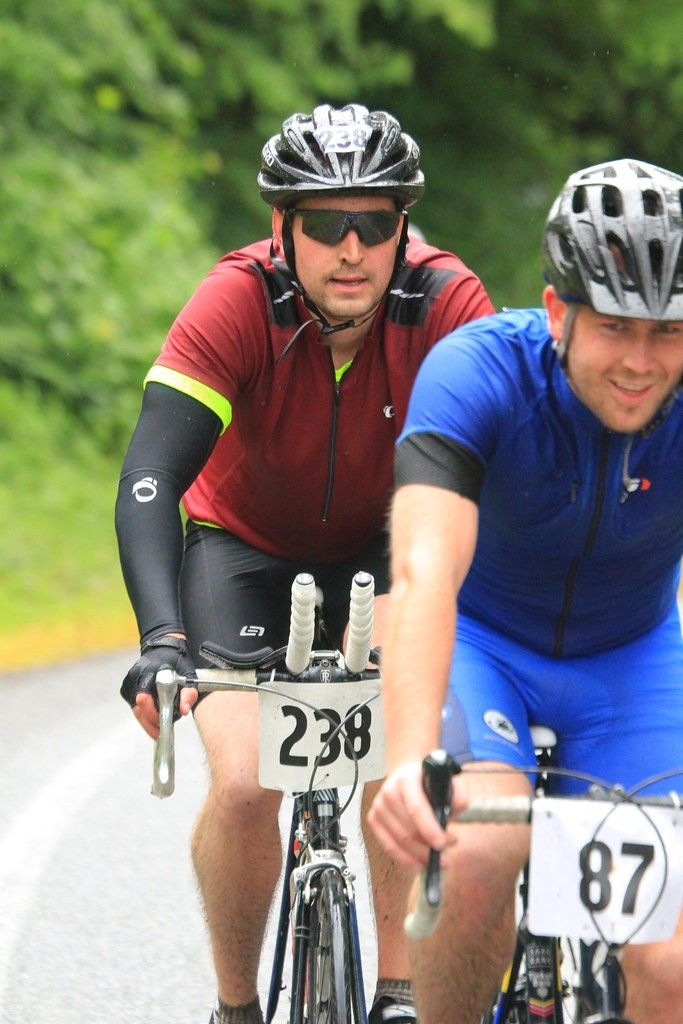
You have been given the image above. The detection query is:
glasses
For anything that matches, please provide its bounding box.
[282,207,408,247]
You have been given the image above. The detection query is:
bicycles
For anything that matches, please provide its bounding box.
[151,570,381,1024]
[422,726,683,1024]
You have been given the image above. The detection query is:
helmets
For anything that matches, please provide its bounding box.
[257,103,425,207]
[541,159,683,321]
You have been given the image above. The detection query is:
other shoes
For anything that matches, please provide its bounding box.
[368,995,416,1024]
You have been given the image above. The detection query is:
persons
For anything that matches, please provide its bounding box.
[114,107,497,1024]
[361,159,683,1024]
